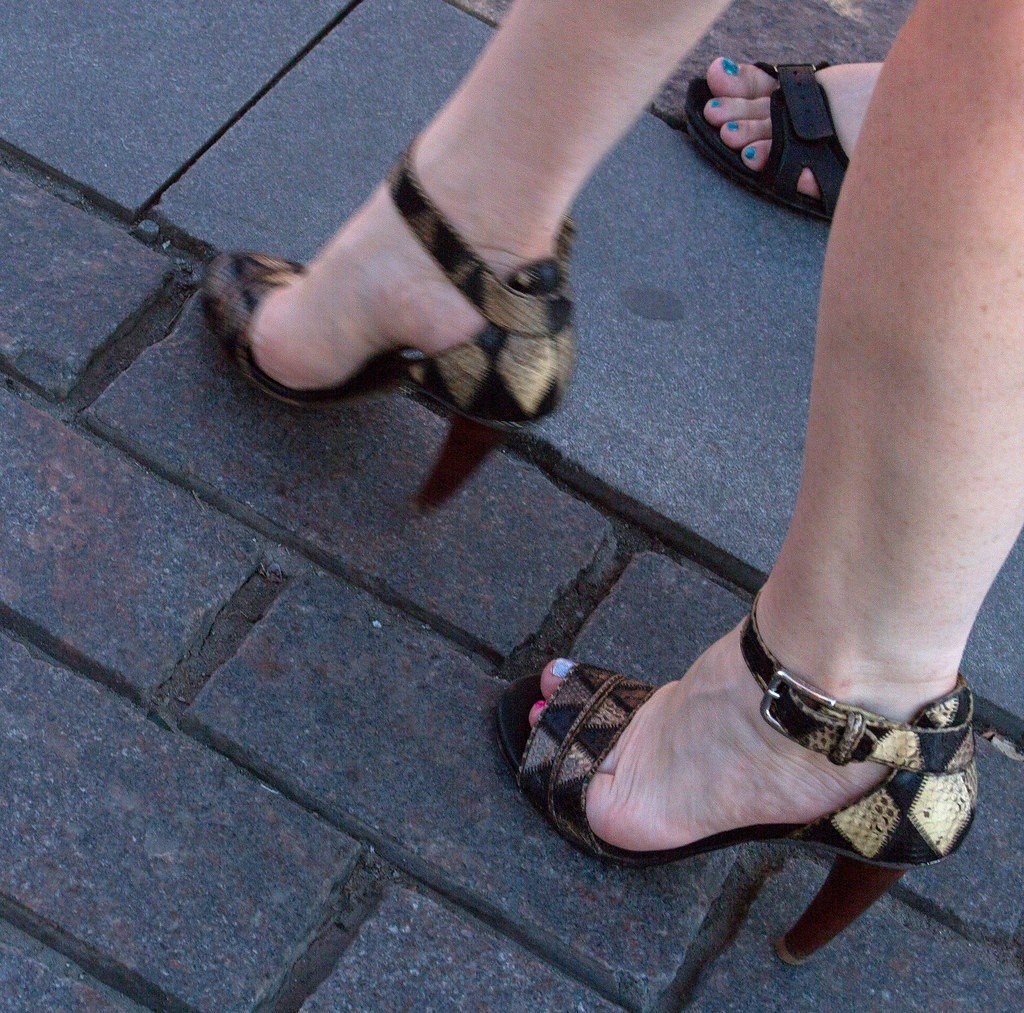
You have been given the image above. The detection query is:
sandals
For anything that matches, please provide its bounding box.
[685,59,851,227]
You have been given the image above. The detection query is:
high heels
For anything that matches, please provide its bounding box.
[493,587,980,968]
[200,137,577,521]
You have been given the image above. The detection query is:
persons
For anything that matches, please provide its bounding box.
[196,0,1024,965]
